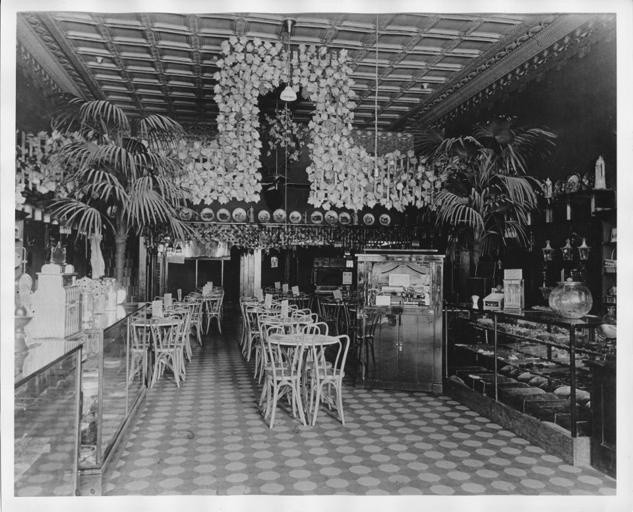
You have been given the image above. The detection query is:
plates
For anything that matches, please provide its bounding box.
[378,214,391,226]
[310,211,323,224]
[363,213,375,225]
[565,172,581,193]
[179,206,247,222]
[338,212,350,225]
[273,208,286,222]
[324,210,338,224]
[257,210,270,222]
[289,210,301,224]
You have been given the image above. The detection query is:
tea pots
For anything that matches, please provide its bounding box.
[246,204,256,222]
[541,177,552,198]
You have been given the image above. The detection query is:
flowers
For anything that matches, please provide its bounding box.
[16,37,447,213]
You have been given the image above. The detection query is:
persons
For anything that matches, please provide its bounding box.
[599,312,616,360]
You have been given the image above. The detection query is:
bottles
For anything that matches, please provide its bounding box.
[79,289,94,322]
[593,155,606,190]
[101,278,116,311]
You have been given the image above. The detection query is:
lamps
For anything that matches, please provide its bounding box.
[279,20,298,105]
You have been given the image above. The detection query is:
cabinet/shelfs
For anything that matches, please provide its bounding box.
[15,333,88,495]
[581,357,617,482]
[70,302,151,496]
[448,314,603,472]
[593,205,616,316]
[472,168,617,310]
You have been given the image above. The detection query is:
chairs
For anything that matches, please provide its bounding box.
[128,270,404,429]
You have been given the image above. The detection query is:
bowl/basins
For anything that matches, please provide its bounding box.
[601,323,617,339]
